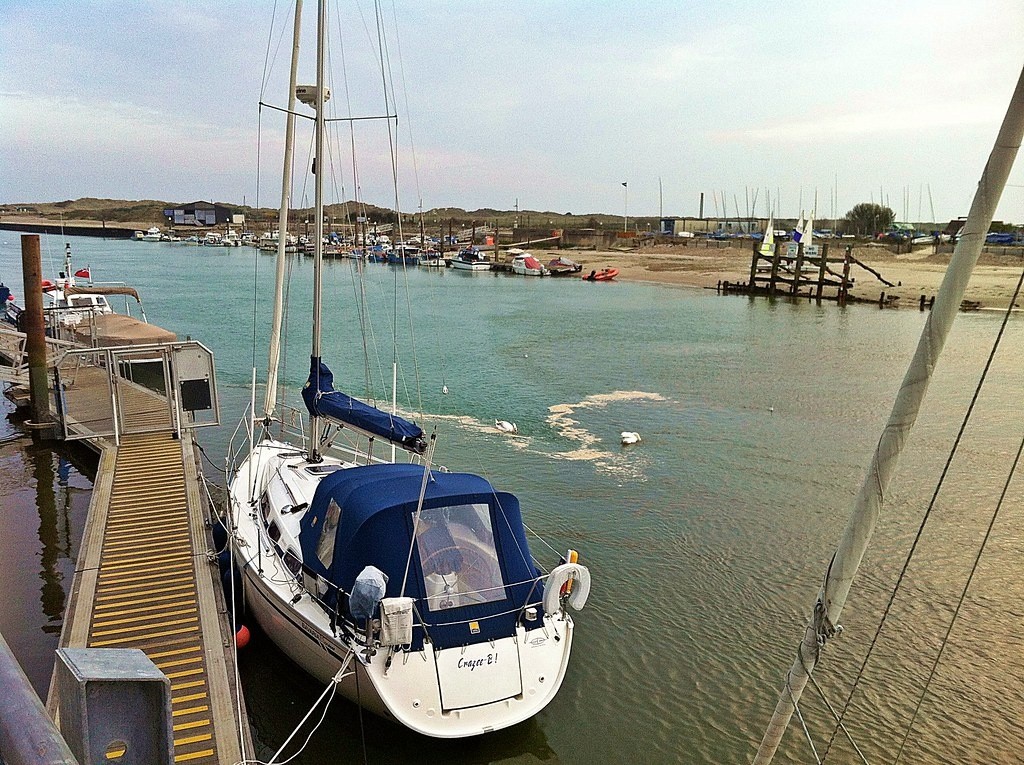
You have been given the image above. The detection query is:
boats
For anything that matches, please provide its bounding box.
[450,246,491,270]
[583,268,619,280]
[131,227,181,243]
[43,211,176,398]
[543,257,582,274]
[185,232,242,247]
[418,248,446,266]
[512,253,551,276]
[388,246,420,264]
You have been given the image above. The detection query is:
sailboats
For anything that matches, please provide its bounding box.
[644,176,671,236]
[212,1,590,739]
[708,173,952,245]
[749,210,822,272]
[258,193,388,262]
